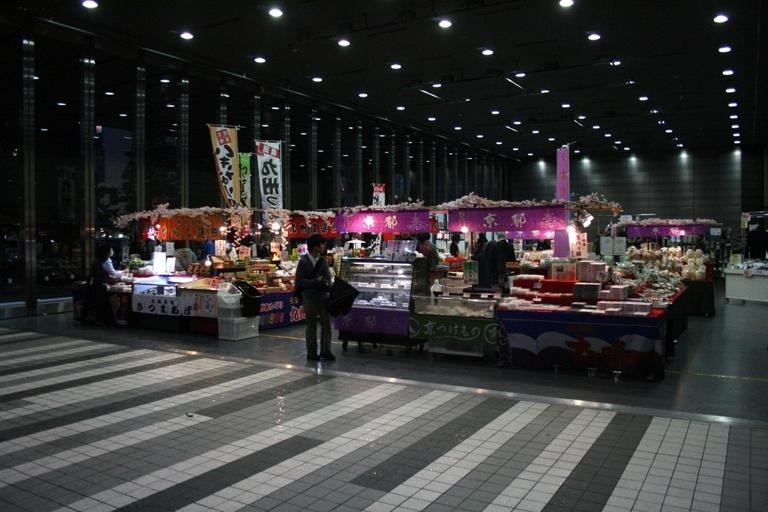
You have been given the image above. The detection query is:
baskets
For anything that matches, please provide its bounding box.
[219,282,242,308]
[217,317,260,340]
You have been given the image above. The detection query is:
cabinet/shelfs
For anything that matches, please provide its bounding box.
[722,260,767,305]
[76,253,314,340]
[333,247,717,384]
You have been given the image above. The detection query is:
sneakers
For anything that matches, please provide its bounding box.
[320,351,335,361]
[116,320,128,325]
[306,354,319,362]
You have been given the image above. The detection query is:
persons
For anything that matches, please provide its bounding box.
[87,245,130,325]
[173,239,197,271]
[416,232,439,265]
[415,236,437,272]
[197,240,217,275]
[496,232,517,262]
[294,233,337,361]
[449,233,460,257]
[477,233,489,249]
